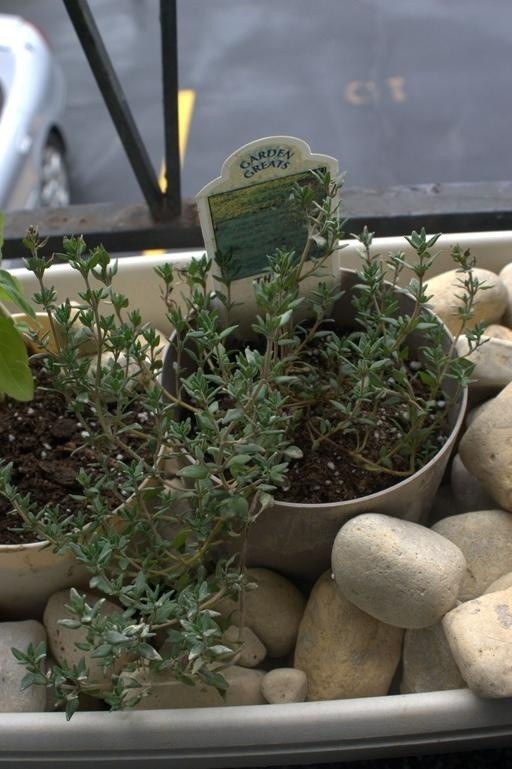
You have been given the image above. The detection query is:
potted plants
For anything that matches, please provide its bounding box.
[0,165,498,616]
